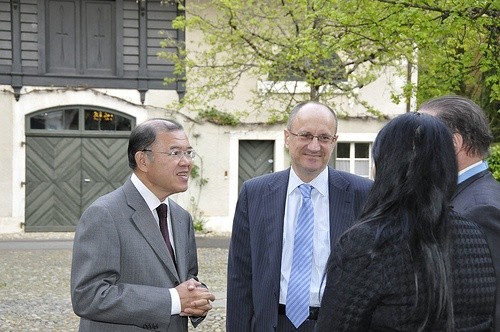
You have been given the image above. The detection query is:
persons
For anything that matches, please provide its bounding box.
[314,112,496,332]
[226,100,375,332]
[417,95,500,332]
[70,118,215,332]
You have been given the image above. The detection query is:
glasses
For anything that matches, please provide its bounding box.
[137,149,195,161]
[287,129,337,145]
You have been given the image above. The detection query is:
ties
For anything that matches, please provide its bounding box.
[156,203,181,280]
[286,183,316,329]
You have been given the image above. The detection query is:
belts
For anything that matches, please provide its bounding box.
[278,303,320,322]
